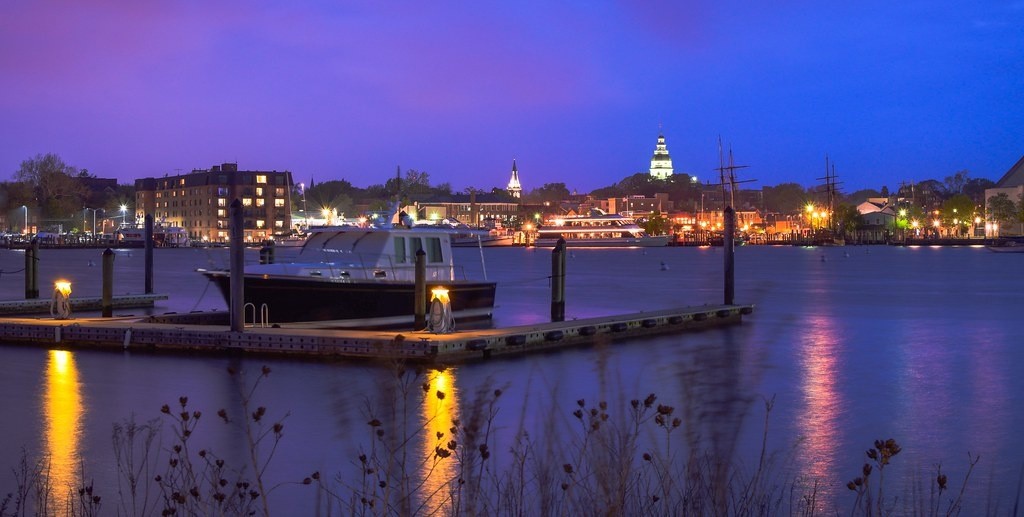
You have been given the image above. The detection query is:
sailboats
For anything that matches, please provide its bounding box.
[814,154,846,247]
[707,136,759,247]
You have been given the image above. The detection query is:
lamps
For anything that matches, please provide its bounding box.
[54,278,72,297]
[431,285,450,305]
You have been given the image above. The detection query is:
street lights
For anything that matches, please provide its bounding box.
[86,207,106,238]
[23,205,27,240]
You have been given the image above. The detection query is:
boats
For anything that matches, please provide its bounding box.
[534,206,673,247]
[193,228,497,328]
[119,216,193,248]
[412,216,512,248]
[984,235,1024,253]
[267,228,307,248]
